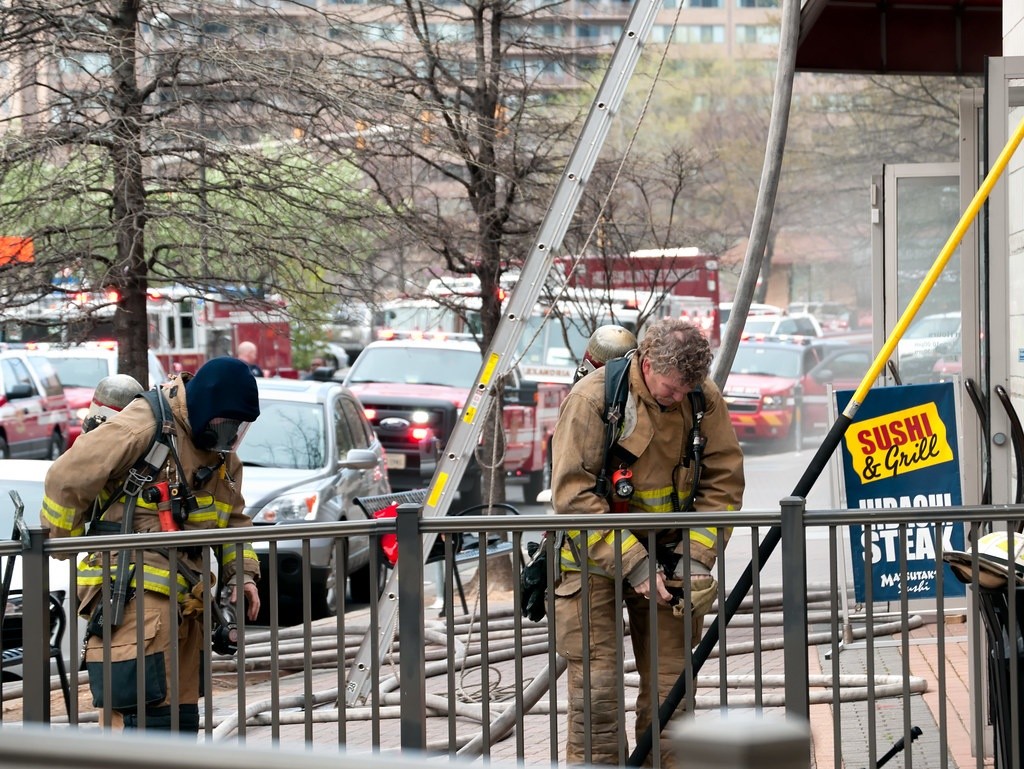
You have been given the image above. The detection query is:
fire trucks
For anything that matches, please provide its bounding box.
[461,247,718,350]
[2,282,298,383]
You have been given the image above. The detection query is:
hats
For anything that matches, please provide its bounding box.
[183,356,260,437]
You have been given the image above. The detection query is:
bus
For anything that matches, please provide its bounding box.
[376,293,642,389]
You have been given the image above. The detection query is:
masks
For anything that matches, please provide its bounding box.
[206,421,241,452]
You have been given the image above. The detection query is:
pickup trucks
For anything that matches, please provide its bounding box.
[344,338,569,517]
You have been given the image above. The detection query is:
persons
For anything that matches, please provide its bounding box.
[236,340,270,377]
[545,316,746,769]
[40,355,261,737]
[302,356,327,383]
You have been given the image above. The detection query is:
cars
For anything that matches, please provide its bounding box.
[236,375,394,625]
[327,302,375,355]
[1,456,232,685]
[705,303,963,449]
[2,350,72,458]
[38,347,165,450]
